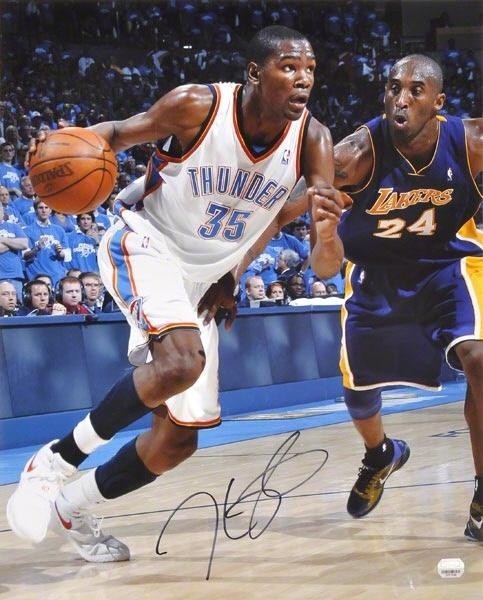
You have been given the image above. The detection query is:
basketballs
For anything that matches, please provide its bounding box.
[28,126,117,214]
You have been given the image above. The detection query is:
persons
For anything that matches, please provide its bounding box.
[197,54,483,541]
[6,26,346,564]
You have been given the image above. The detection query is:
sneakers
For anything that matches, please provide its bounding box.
[6,436,80,546]
[464,496,483,545]
[45,489,132,564]
[345,439,411,520]
